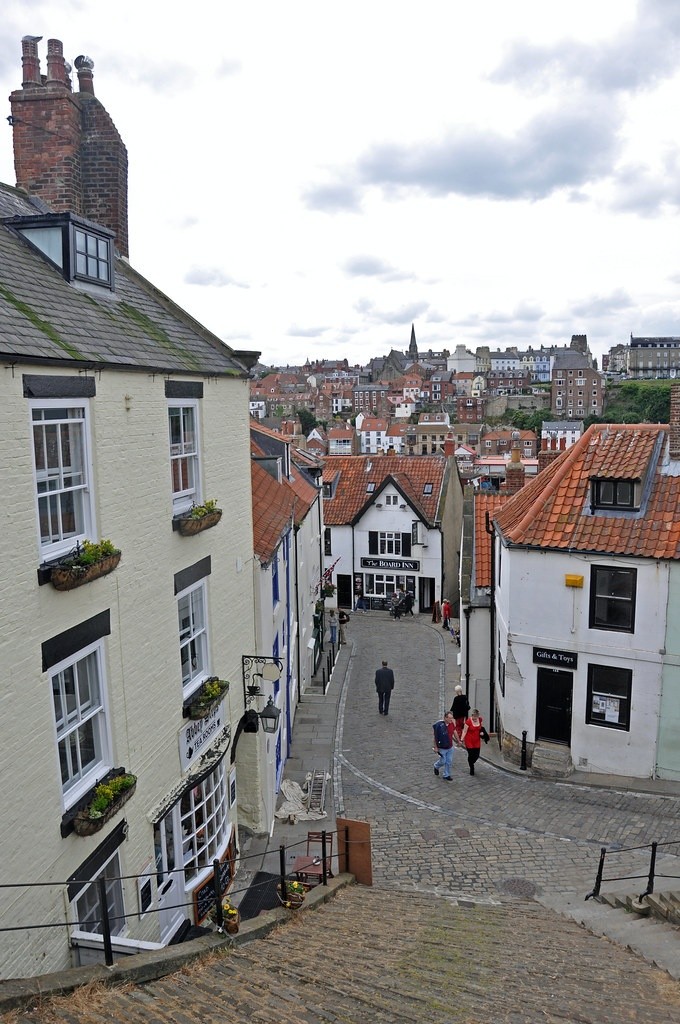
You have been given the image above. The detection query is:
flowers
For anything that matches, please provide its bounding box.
[289,881,306,896]
[220,896,237,920]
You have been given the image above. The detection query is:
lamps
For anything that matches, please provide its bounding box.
[243,694,281,734]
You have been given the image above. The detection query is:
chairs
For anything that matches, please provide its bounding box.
[306,831,332,879]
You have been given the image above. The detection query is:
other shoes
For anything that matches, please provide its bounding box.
[443,776,452,781]
[384,710,387,715]
[434,766,439,775]
[379,710,383,713]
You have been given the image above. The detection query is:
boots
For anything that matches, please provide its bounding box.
[470,763,474,775]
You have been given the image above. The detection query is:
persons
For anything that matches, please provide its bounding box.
[328,609,337,644]
[337,608,350,644]
[441,599,451,631]
[351,584,367,613]
[390,588,414,620]
[432,711,460,781]
[451,685,471,747]
[461,708,484,775]
[375,660,395,716]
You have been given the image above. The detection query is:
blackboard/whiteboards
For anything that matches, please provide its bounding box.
[192,850,234,925]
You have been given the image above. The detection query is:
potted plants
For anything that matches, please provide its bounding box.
[49,539,121,591]
[73,771,138,835]
[189,676,229,720]
[180,499,222,536]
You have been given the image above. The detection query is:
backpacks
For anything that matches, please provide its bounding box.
[342,613,350,622]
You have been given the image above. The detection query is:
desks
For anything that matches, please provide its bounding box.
[293,855,323,884]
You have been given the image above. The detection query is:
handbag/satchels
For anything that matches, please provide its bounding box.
[465,696,470,710]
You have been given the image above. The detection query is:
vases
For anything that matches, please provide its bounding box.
[220,914,242,933]
[287,892,305,909]
